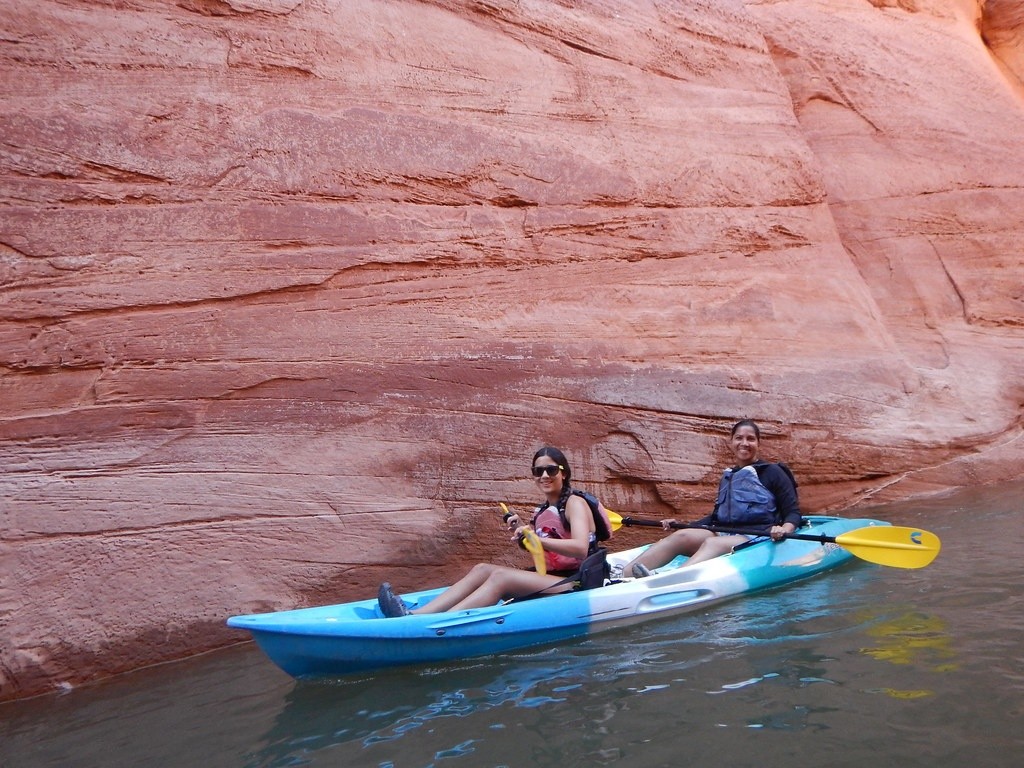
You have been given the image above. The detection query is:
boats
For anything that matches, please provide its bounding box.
[225,510,892,683]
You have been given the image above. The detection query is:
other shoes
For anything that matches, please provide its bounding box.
[385,595,413,618]
[377,582,394,615]
[631,562,658,579]
[609,564,625,578]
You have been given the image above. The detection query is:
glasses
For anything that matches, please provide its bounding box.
[531,465,565,477]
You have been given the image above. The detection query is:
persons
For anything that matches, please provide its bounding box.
[376,445,612,616]
[603,419,802,586]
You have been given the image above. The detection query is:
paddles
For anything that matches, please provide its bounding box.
[499,501,546,576]
[604,509,941,570]
